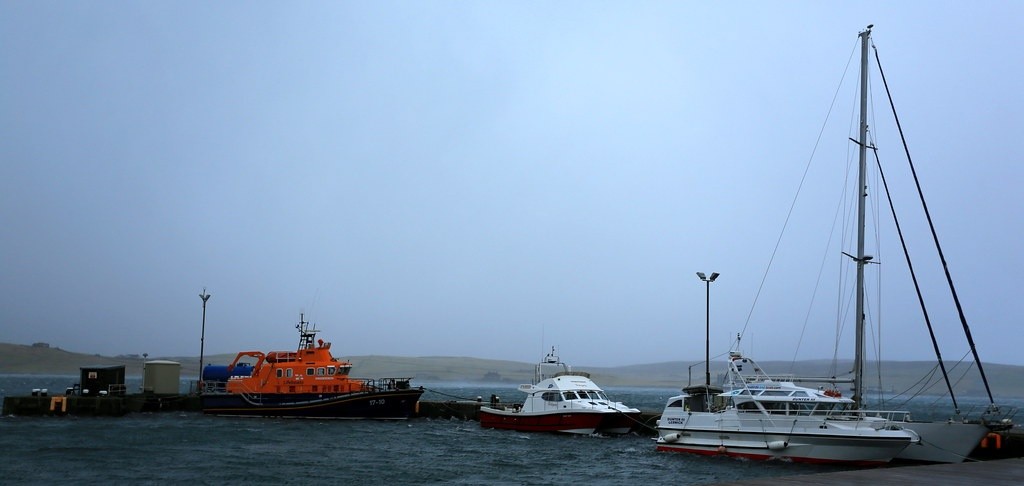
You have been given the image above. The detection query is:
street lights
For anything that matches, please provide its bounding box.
[480,344,643,436]
[695,271,721,384]
[199,287,211,380]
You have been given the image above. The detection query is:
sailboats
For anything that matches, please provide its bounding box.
[721,19,1016,467]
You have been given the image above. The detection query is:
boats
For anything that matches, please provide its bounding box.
[197,312,426,422]
[652,332,928,472]
